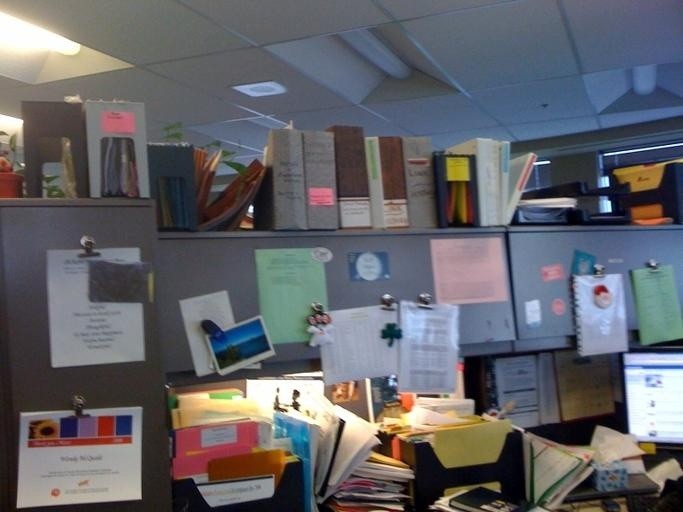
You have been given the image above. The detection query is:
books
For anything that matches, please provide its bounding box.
[169,370,647,511]
[147,126,537,232]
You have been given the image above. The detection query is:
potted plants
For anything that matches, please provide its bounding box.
[0,130,65,197]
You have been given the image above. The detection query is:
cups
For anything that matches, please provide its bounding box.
[101,136,139,199]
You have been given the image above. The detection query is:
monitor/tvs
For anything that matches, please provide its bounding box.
[618,348,683,450]
